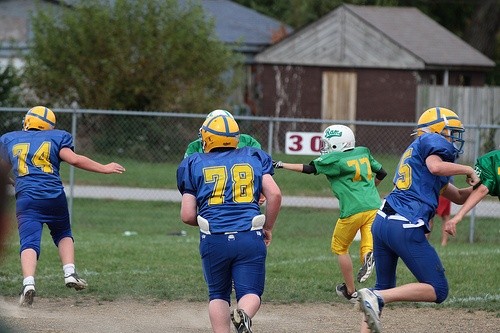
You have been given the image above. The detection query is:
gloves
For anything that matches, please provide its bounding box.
[271,160,283,169]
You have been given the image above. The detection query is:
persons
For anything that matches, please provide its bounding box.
[176,115,281,333]
[350,107,480,333]
[425,196,451,246]
[0,106,125,307]
[184,109,265,206]
[445,148,500,236]
[273,124,387,303]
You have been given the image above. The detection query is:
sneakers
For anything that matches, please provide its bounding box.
[230,309,253,333]
[64,270,88,291]
[18,284,36,306]
[357,252,375,283]
[357,288,385,333]
[335,281,358,304]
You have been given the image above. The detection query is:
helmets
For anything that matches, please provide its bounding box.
[206,109,234,120]
[320,124,355,155]
[201,115,240,153]
[417,107,464,142]
[24,106,56,130]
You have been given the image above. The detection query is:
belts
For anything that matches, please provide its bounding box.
[376,210,424,228]
